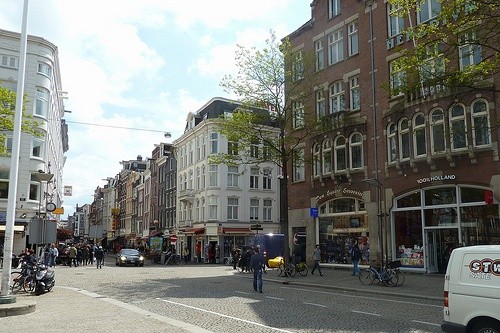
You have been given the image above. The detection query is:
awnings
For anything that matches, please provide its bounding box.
[224,228,252,233]
[182,228,204,234]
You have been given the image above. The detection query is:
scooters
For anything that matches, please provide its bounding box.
[34,265,56,296]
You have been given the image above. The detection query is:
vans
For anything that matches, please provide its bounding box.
[441,245,500,333]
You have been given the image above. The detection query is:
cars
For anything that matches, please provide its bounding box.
[115,248,144,267]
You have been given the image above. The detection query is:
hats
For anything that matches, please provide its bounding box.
[99,245,103,249]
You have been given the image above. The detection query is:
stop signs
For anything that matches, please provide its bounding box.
[170,235,177,242]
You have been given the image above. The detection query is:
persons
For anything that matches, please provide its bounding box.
[184,247,189,263]
[232,248,254,272]
[45,243,99,267]
[311,245,324,275]
[352,244,363,276]
[23,249,36,286]
[96,246,103,269]
[251,248,264,292]
[25,248,30,255]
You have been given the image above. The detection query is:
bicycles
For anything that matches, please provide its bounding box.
[358,256,405,287]
[11,261,39,293]
[272,253,308,277]
